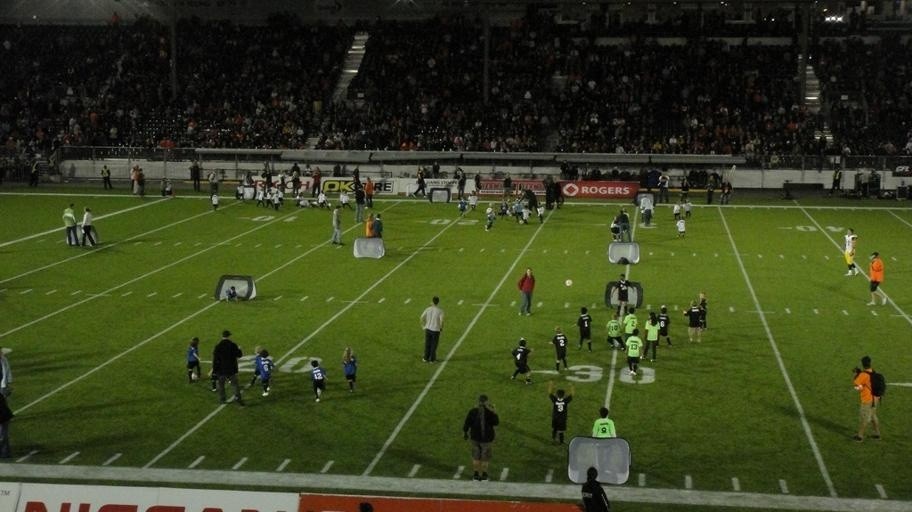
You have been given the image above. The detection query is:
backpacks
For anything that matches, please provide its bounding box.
[869,370,884,398]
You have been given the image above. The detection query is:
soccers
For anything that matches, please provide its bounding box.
[565,280,571,286]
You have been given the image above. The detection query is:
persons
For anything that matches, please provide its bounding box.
[552,325,568,371]
[510,340,533,385]
[676,218,687,239]
[463,394,499,481]
[852,356,880,442]
[419,296,444,364]
[1,2,911,187]
[518,268,535,316]
[188,159,200,191]
[606,292,707,375]
[459,189,477,215]
[843,228,860,275]
[226,287,239,304]
[340,167,373,221]
[332,205,344,244]
[671,203,680,218]
[484,189,545,232]
[61,203,80,245]
[682,200,694,218]
[612,274,634,317]
[130,162,145,195]
[158,177,174,196]
[866,252,887,306]
[610,207,633,244]
[367,211,383,240]
[548,380,616,512]
[187,336,201,382]
[0,355,13,458]
[235,162,327,210]
[577,308,593,352]
[640,195,654,227]
[208,169,220,198]
[80,207,97,248]
[101,164,112,189]
[342,347,357,392]
[210,331,274,403]
[652,175,733,204]
[311,361,328,402]
